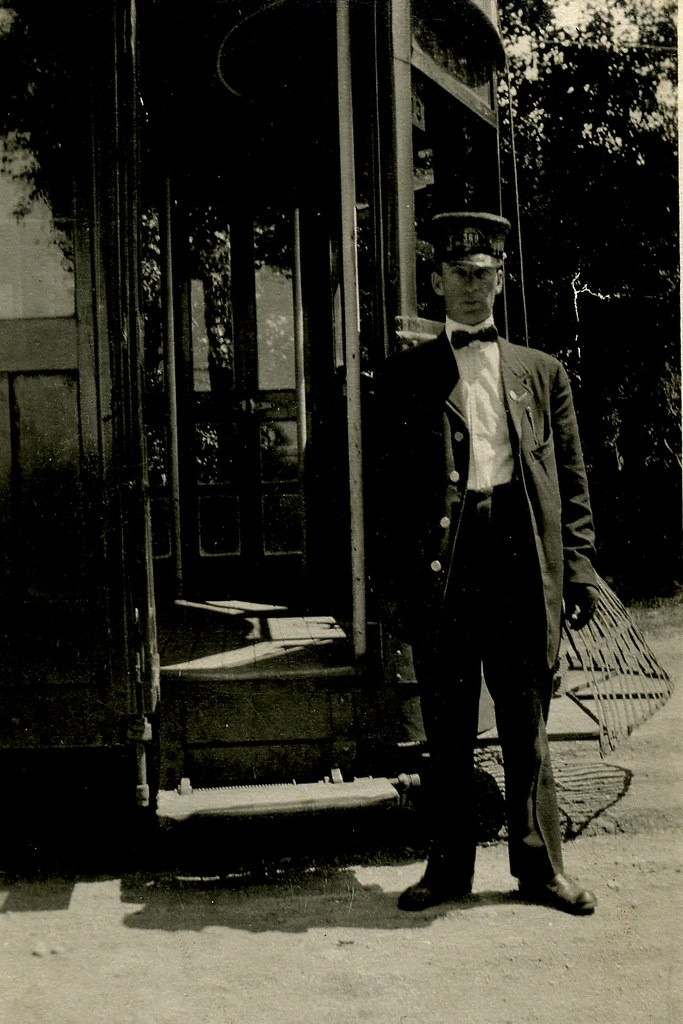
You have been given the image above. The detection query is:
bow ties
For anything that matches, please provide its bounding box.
[451,326,499,351]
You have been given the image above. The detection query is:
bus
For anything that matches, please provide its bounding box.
[0,0,505,806]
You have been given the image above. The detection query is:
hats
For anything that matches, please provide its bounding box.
[430,214,512,270]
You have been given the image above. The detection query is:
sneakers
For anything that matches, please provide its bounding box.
[397,873,469,912]
[520,875,597,912]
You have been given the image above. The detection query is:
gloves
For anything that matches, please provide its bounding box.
[563,584,599,631]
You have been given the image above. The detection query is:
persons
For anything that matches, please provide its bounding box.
[370,213,599,915]
